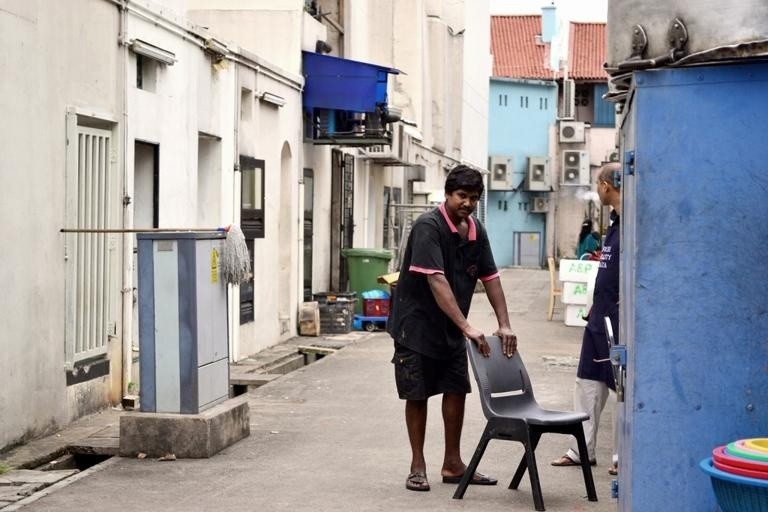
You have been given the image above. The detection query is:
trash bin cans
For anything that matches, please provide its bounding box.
[341,248,392,315]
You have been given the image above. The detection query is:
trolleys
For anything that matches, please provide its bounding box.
[354,313,389,332]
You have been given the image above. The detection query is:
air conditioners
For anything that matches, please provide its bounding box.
[486,119,592,214]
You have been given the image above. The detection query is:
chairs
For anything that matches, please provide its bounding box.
[450,331,601,509]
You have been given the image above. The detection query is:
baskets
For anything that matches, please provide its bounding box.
[314,291,357,335]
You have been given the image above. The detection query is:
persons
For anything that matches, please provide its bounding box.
[384,162,519,491]
[549,160,619,476]
[574,219,601,261]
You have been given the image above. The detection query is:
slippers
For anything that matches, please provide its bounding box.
[404,470,430,492]
[441,466,497,485]
[550,453,598,466]
